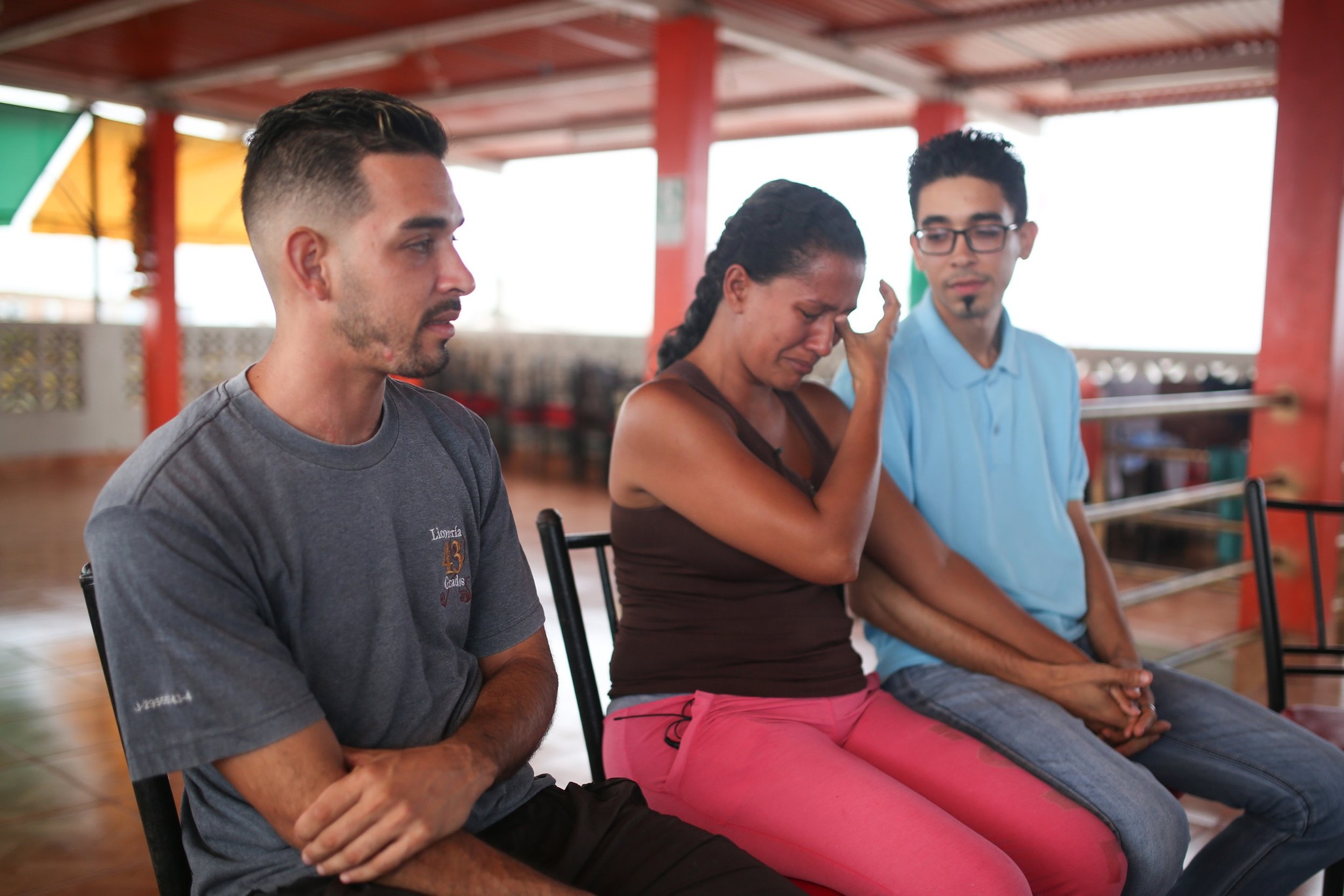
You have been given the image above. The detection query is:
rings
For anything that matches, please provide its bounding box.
[1139,703,1156,711]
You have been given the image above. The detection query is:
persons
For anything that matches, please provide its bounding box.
[828,130,1344,896]
[604,178,1129,896]
[87,87,804,896]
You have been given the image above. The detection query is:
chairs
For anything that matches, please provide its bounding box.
[1246,478,1344,894]
[538,509,618,784]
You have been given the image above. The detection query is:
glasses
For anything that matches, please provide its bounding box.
[914,220,1027,256]
